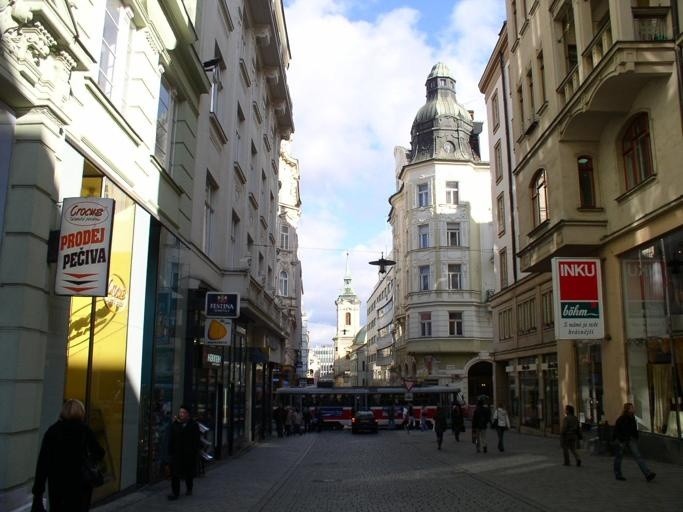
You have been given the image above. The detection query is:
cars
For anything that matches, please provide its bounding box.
[351,411,378,434]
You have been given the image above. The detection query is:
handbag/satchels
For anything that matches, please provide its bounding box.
[490,419,498,428]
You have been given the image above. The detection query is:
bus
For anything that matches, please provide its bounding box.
[276,381,471,430]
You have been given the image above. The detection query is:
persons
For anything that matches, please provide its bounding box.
[162,402,202,501]
[273,404,322,438]
[610,403,656,482]
[561,405,582,467]
[386,394,510,453]
[31,398,96,512]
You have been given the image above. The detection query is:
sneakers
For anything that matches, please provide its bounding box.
[576,459,581,466]
[646,472,656,482]
[616,476,626,481]
[477,447,487,453]
[563,463,570,466]
[497,446,503,452]
[168,489,193,499]
